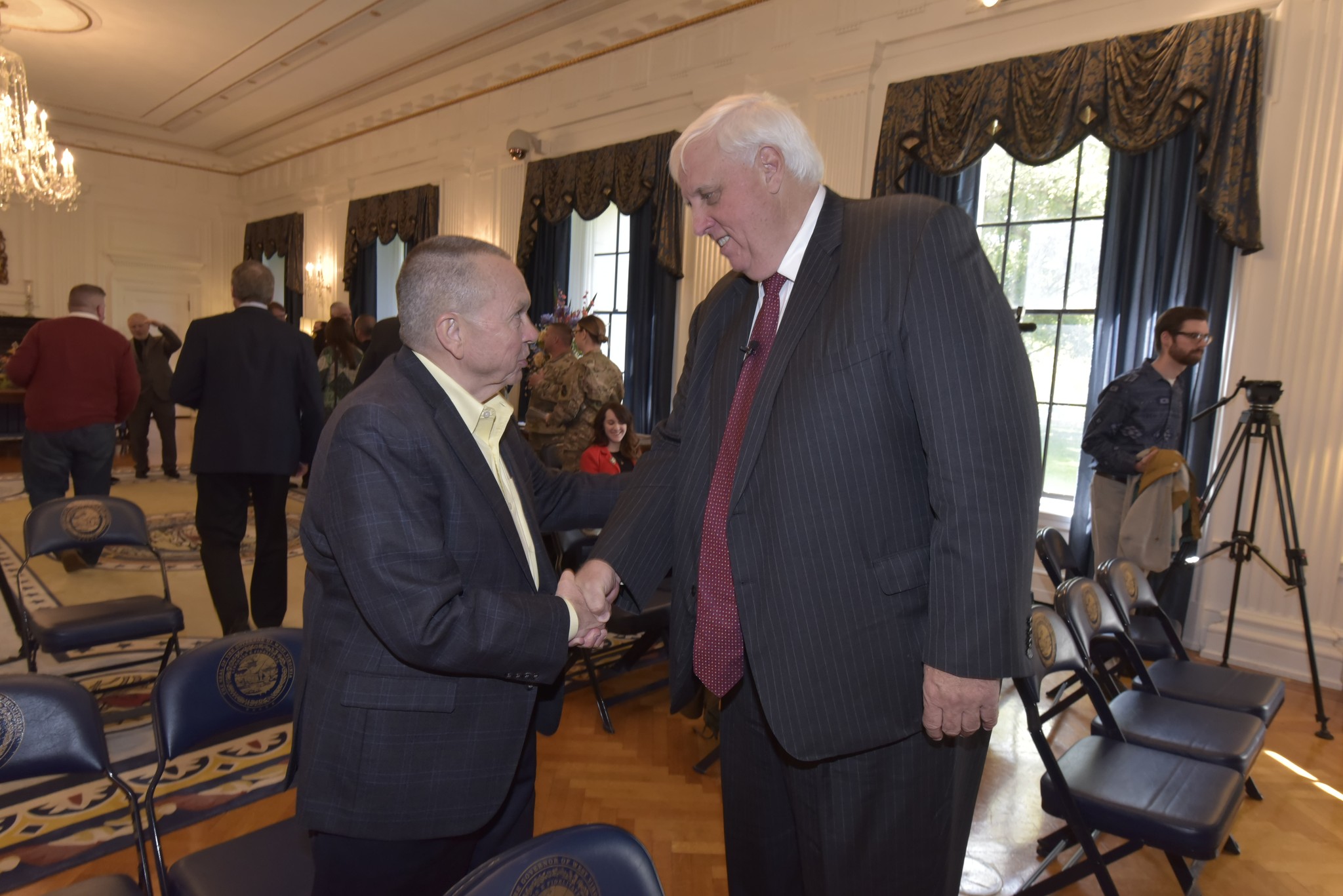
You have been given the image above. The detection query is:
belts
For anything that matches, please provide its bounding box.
[1095,470,1128,484]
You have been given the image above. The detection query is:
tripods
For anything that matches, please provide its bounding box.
[1156,376,1335,741]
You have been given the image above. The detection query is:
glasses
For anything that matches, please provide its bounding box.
[1171,331,1214,346]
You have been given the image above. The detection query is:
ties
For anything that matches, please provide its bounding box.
[694,271,796,698]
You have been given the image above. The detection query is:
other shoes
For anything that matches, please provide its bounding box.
[134,470,147,479]
[164,469,180,478]
[61,549,88,572]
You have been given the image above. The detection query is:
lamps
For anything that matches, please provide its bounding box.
[306,256,333,296]
[0,47,81,213]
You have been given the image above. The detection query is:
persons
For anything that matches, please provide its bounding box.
[5,284,140,573]
[571,94,1043,896]
[280,235,604,896]
[526,317,646,651]
[1081,305,1213,701]
[110,313,182,485]
[268,259,401,489]
[171,260,326,636]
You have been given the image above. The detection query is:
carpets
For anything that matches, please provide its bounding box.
[0,463,670,893]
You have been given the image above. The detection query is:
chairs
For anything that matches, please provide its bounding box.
[551,529,675,733]
[16,494,184,672]
[1054,573,1267,853]
[1097,557,1284,800]
[1013,600,1244,896]
[1037,525,1184,719]
[444,822,666,896]
[143,627,316,895]
[0,673,153,895]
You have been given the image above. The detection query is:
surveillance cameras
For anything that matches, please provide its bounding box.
[507,130,531,160]
